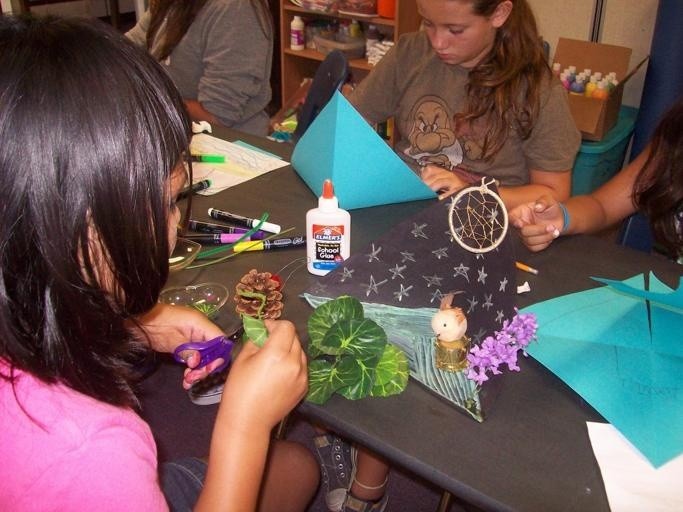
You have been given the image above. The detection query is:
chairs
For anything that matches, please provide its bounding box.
[293,48,349,144]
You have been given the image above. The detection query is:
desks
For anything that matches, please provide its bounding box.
[122,116,683,511]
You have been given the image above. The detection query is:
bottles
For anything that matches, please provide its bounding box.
[305,179,350,275]
[550,60,619,98]
[288,15,377,60]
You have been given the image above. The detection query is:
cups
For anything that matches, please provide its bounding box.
[376,0,395,21]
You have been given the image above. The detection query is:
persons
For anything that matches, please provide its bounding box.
[124,0,277,138]
[508,97,683,267]
[0,12,321,512]
[310,0,583,512]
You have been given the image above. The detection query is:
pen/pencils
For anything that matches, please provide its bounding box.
[515,261,539,276]
[176,179,212,202]
[183,207,306,253]
[185,155,225,163]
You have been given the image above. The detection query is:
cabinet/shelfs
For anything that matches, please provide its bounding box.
[279,1,419,151]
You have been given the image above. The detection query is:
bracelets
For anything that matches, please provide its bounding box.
[559,203,570,234]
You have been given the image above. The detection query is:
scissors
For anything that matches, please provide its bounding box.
[173,315,257,379]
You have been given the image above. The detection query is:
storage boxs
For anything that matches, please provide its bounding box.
[550,36,650,141]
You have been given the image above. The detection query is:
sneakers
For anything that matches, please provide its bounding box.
[312,429,389,512]
